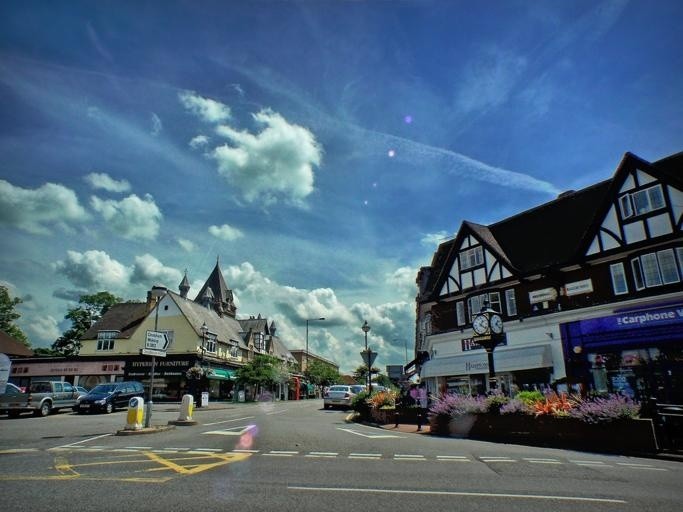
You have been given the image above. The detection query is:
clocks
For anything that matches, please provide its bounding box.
[490,315,503,334]
[473,314,489,335]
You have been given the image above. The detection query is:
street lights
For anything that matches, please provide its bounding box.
[306,318,325,398]
[196,321,209,407]
[362,319,371,397]
[393,338,408,375]
[141,286,168,430]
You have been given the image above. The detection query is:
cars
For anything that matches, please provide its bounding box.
[6,382,24,393]
[74,386,89,396]
[323,382,389,409]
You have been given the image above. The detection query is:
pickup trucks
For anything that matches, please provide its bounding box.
[0,381,88,416]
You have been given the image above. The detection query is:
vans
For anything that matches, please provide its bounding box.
[76,380,145,413]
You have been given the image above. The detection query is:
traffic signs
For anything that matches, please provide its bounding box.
[146,329,168,349]
[141,348,166,358]
